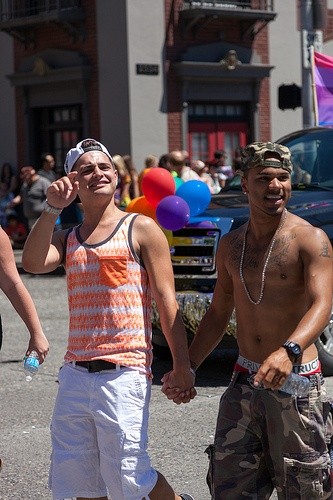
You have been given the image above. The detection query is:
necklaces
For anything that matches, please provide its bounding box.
[240,205,287,305]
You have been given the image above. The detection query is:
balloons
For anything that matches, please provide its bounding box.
[127,167,211,248]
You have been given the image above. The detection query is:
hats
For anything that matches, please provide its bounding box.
[240,141,294,176]
[20,166,35,179]
[64,138,113,175]
[191,160,205,172]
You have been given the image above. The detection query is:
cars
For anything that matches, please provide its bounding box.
[153,127,333,377]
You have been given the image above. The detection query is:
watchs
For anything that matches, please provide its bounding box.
[281,341,303,364]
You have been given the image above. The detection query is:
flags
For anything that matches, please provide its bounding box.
[312,53,333,126]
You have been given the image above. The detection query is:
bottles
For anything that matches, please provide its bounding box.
[23,350,40,381]
[277,373,310,397]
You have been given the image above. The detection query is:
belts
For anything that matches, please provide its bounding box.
[236,372,325,392]
[75,359,125,374]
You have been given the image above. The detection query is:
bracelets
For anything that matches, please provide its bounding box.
[43,200,62,216]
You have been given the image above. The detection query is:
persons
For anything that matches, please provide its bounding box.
[0,220,49,366]
[23,139,197,500]
[220,50,241,71]
[160,140,333,500]
[0,150,313,238]
